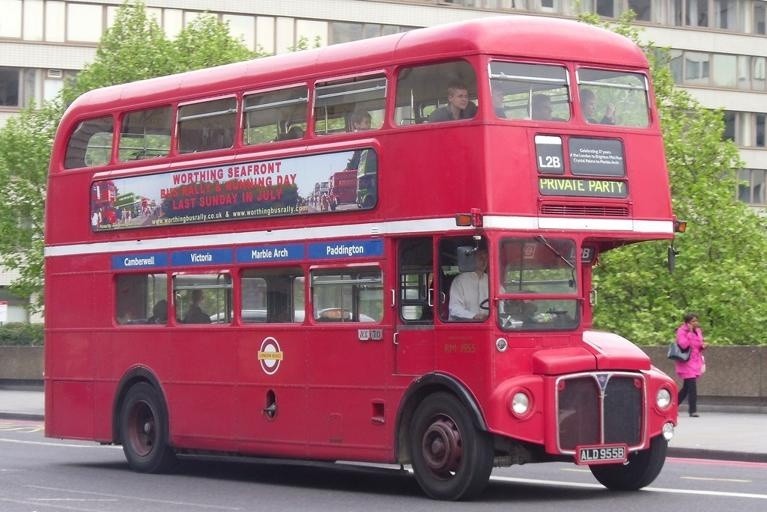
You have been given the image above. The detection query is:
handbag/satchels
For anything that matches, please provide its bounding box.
[667,342,691,361]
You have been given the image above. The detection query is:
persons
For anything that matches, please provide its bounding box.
[492,85,508,118]
[429,81,477,122]
[147,299,167,324]
[183,289,212,324]
[674,315,706,416]
[351,109,371,131]
[288,126,304,139]
[528,94,567,121]
[581,88,615,124]
[448,245,506,322]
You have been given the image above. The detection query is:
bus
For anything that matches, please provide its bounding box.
[43,14,687,501]
